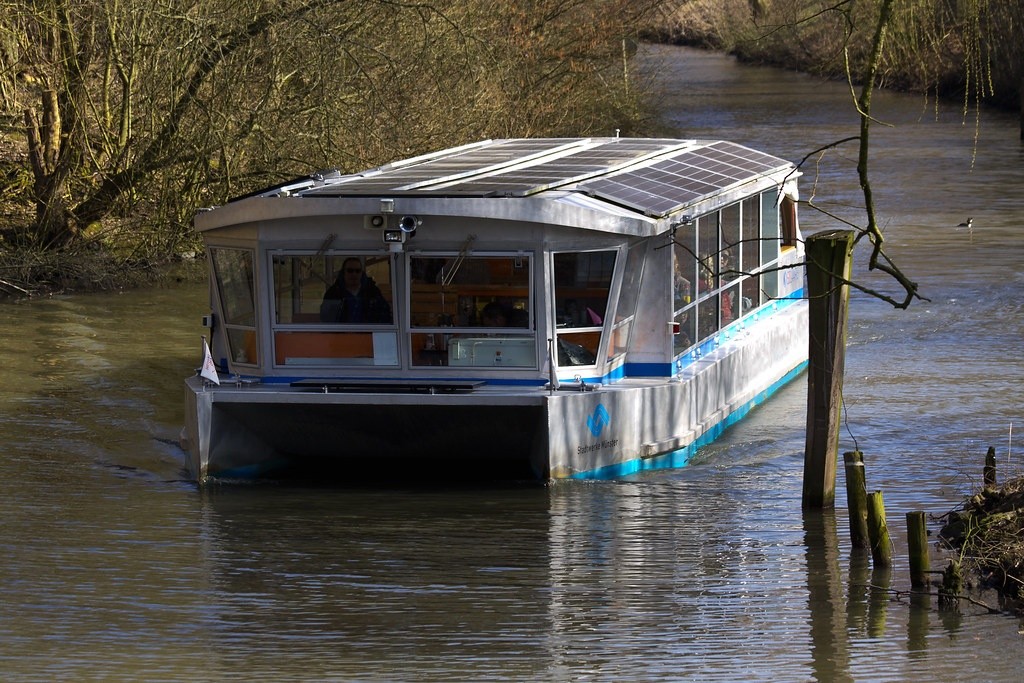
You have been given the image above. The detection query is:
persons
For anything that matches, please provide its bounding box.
[481,302,535,337]
[565,297,602,326]
[320,257,393,323]
[617,243,738,345]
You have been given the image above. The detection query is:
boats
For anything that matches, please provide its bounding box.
[183,127,811,491]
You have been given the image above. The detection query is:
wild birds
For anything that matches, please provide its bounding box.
[958,217,974,228]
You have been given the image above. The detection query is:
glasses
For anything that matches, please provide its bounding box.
[347,268,362,273]
[721,253,728,259]
